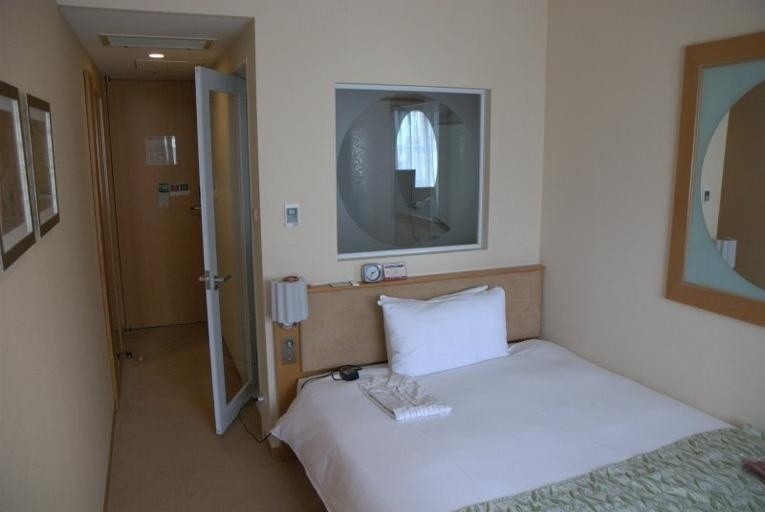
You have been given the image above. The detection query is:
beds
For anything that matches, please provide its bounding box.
[270,264,765,512]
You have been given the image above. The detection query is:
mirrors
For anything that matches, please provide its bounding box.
[334,83,491,262]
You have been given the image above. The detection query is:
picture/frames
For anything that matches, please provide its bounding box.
[664,31,765,327]
[21,93,59,238]
[0,80,36,271]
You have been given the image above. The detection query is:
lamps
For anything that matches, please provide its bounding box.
[271,275,310,329]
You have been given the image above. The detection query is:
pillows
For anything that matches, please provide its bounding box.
[377,286,509,375]
[380,285,487,367]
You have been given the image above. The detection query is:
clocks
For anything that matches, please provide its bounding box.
[362,264,384,283]
